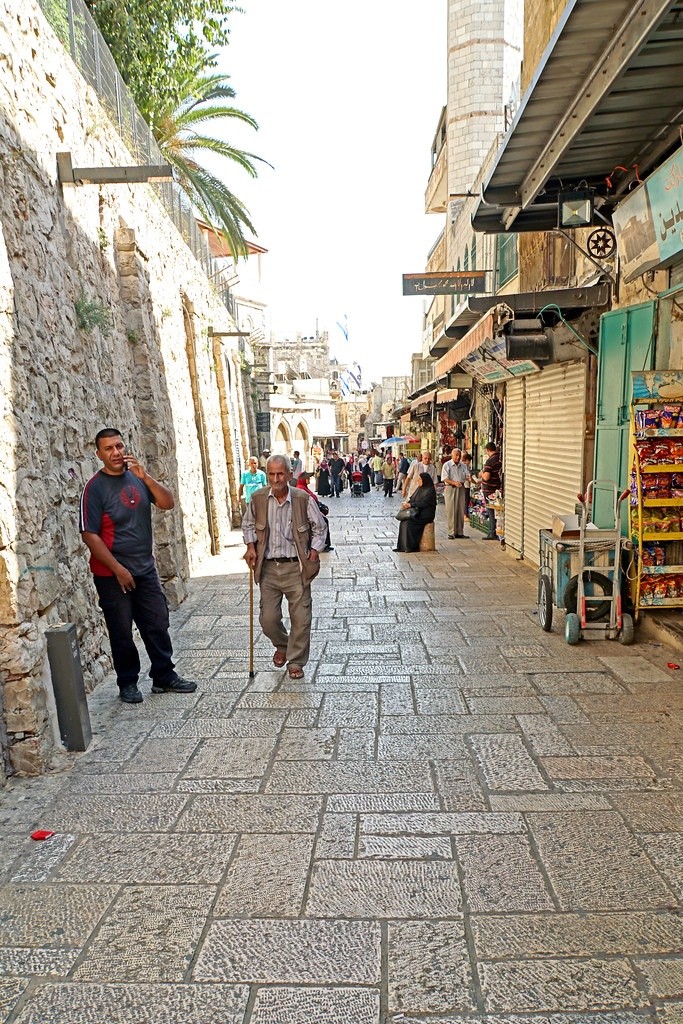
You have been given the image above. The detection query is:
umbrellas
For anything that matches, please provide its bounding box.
[379,435,420,449]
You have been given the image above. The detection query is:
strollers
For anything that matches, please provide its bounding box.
[350,471,364,498]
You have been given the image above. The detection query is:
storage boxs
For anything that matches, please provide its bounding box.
[552,514,600,540]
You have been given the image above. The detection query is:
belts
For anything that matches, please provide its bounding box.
[267,557,299,562]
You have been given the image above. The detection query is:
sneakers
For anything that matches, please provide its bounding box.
[120,686,143,703]
[151,675,197,693]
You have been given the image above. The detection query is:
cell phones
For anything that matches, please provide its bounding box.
[125,445,129,471]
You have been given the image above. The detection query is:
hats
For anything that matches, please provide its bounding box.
[298,471,315,480]
[263,449,271,453]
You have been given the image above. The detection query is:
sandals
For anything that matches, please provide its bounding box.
[290,669,304,679]
[273,650,286,667]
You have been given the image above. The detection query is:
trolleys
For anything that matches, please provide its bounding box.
[534,478,636,647]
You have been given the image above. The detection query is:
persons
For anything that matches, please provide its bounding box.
[241,455,328,679]
[78,429,198,703]
[477,442,503,540]
[238,456,268,510]
[259,450,271,474]
[287,450,472,552]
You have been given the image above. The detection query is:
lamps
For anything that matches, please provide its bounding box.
[557,189,593,228]
[506,320,549,360]
[446,372,473,388]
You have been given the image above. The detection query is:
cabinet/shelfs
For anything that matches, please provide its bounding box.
[627,370,683,621]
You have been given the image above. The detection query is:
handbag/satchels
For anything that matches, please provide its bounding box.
[313,467,321,478]
[317,501,329,516]
[396,507,418,519]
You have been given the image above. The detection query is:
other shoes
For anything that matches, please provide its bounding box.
[482,534,496,540]
[456,533,469,538]
[389,494,393,498]
[392,548,400,552]
[321,547,334,553]
[384,493,387,497]
[447,535,453,539]
